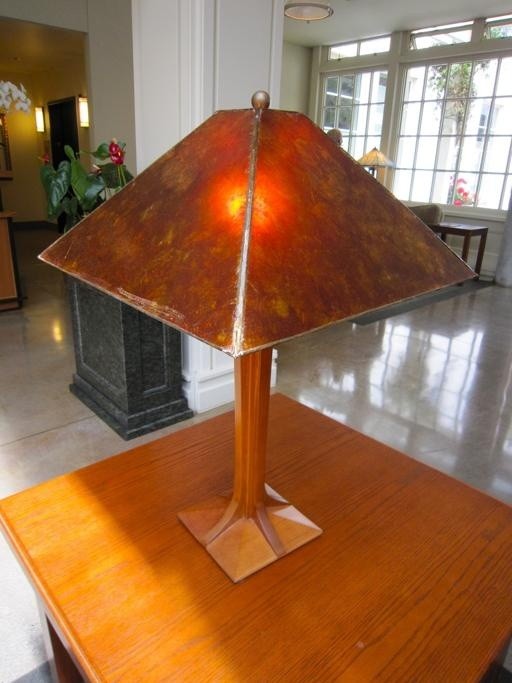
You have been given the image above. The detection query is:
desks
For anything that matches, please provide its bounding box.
[423,220,488,284]
[2,382,509,683]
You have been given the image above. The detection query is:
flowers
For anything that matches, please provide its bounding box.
[36,131,143,231]
[0,78,31,120]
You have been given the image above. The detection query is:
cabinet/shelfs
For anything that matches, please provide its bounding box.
[0,208,26,312]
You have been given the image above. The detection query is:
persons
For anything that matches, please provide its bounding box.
[326,128,343,147]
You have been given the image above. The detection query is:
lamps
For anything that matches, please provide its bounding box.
[281,0,334,26]
[35,83,479,588]
[35,103,45,133]
[354,142,393,180]
[76,92,91,128]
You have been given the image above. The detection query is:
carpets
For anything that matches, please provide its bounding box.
[345,269,494,326]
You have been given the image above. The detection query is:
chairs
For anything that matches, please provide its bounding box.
[399,203,444,240]
